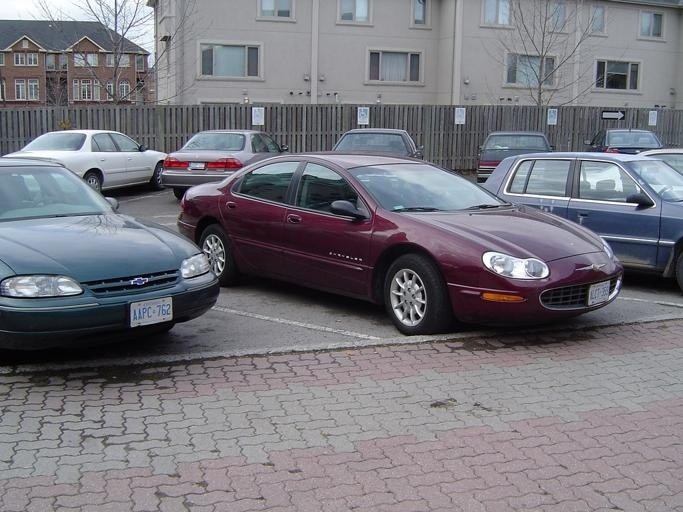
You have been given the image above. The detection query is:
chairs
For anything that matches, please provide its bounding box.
[596,180,627,199]
[580,181,603,200]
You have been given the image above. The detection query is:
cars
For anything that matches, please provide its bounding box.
[1,156,220,351]
[178,149,624,336]
[161,129,290,200]
[481,152,682,290]
[477,133,554,183]
[8,131,170,194]
[333,129,424,163]
[584,127,683,177]
